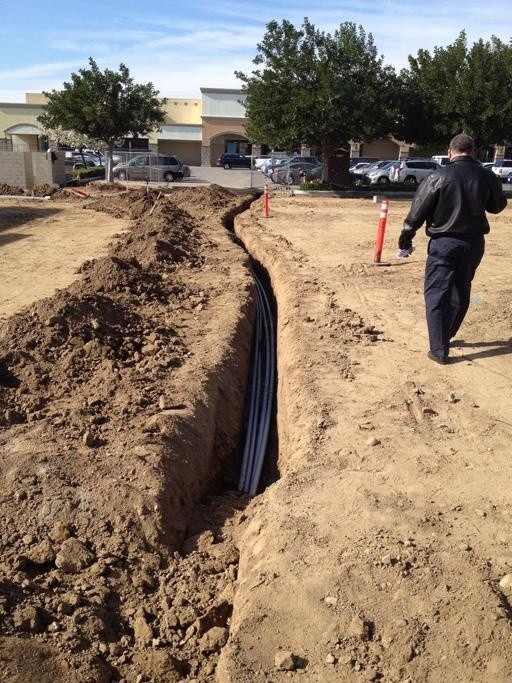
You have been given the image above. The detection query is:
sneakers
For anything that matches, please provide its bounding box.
[428,351,448,365]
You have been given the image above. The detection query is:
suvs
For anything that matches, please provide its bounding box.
[113,154,180,182]
[389,160,443,185]
[217,153,255,169]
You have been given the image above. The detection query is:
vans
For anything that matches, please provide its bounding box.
[431,156,450,168]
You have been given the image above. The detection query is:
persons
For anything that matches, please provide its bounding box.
[398,134,507,366]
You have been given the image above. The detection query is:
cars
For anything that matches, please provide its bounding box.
[348,161,399,185]
[65,148,121,171]
[483,159,512,183]
[253,156,324,185]
[172,156,190,179]
